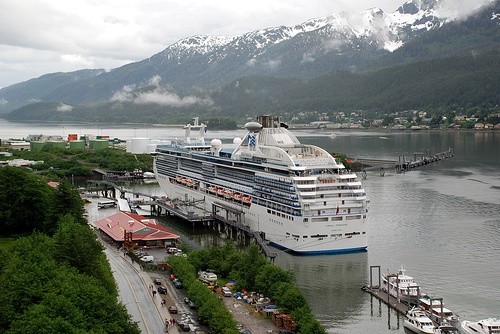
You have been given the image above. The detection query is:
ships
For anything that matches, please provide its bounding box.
[149,113,372,257]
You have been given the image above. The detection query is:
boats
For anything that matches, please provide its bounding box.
[224,190,234,200]
[380,263,426,307]
[143,171,155,178]
[410,125,421,130]
[242,195,251,205]
[185,178,193,187]
[217,188,225,196]
[208,186,217,195]
[389,124,407,130]
[233,193,242,201]
[402,291,500,334]
[97,199,118,209]
[176,176,187,185]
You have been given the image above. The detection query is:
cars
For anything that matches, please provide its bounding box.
[168,306,177,314]
[197,268,218,288]
[168,274,183,289]
[158,286,167,294]
[130,246,188,263]
[174,296,200,334]
[154,278,161,285]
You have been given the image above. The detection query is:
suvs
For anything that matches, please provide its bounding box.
[221,287,232,297]
